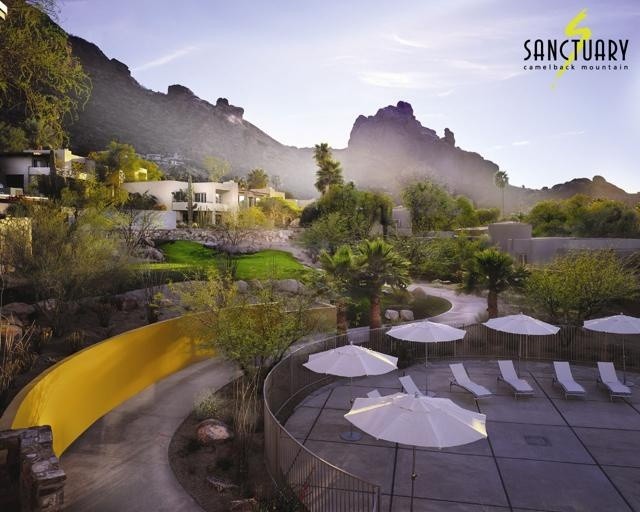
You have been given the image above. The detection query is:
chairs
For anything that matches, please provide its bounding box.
[367,361,632,403]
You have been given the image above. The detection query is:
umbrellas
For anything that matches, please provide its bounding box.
[344,391,487,512]
[582,312,640,385]
[302,340,398,437]
[481,312,561,378]
[386,318,466,395]
